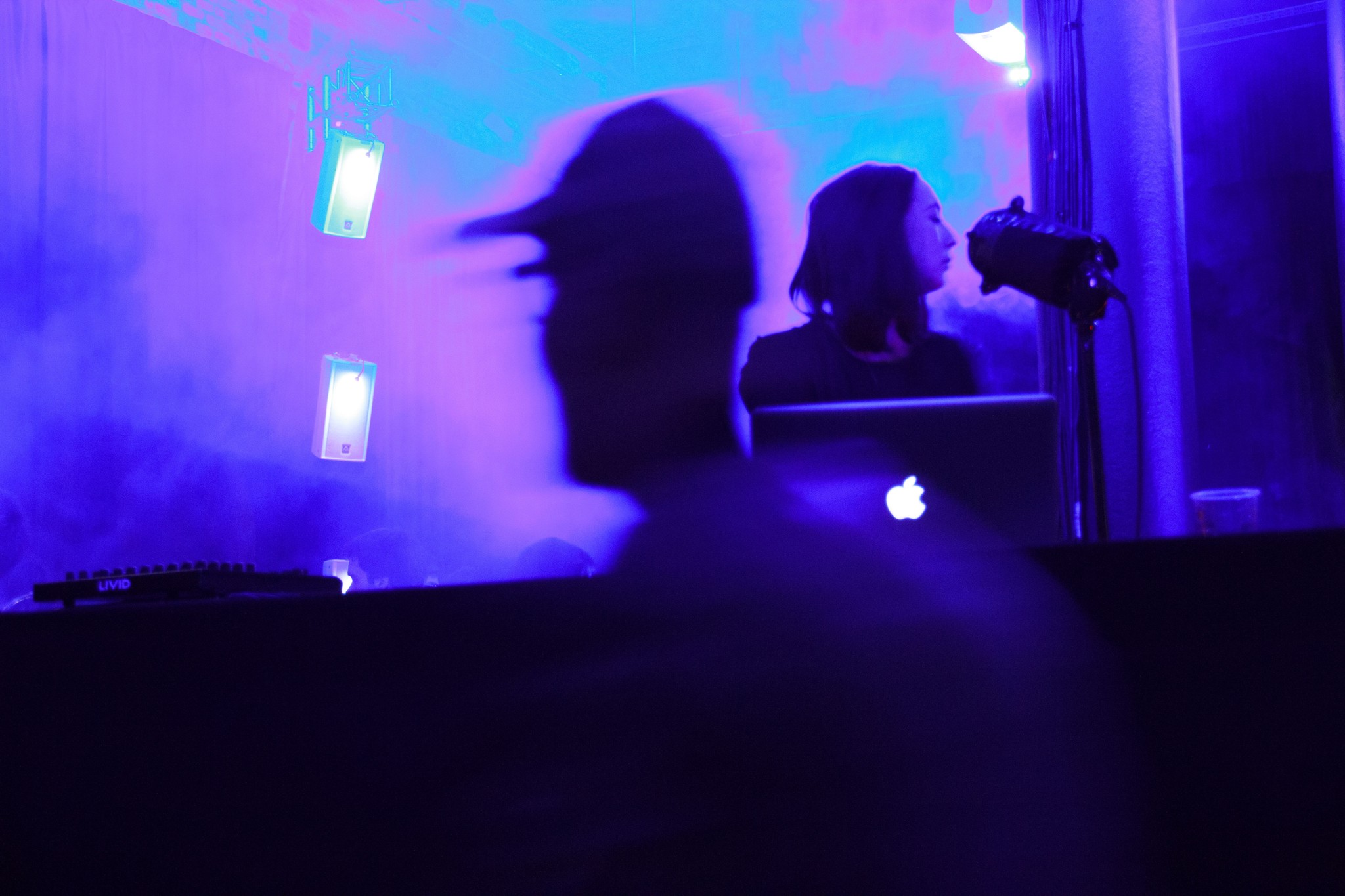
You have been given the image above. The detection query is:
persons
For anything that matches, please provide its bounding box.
[739,163,983,410]
[441,99,1126,896]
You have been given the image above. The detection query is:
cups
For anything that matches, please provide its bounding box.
[1191,488,1263,536]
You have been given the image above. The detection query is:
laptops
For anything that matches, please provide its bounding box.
[749,391,1057,559]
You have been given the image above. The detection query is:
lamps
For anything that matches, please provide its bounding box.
[954,0,1025,66]
